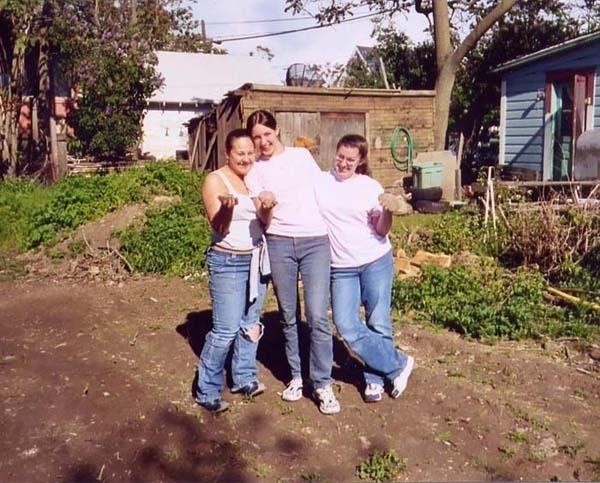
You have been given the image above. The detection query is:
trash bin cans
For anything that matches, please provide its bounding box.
[411,162,443,190]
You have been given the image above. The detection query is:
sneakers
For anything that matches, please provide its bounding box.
[364,382,384,402]
[312,384,341,414]
[282,379,303,402]
[198,399,228,414]
[392,354,414,397]
[232,379,266,397]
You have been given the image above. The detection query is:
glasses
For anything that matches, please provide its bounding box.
[335,156,359,166]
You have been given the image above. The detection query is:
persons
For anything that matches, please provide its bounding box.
[321,132,415,402]
[195,126,270,411]
[244,108,341,414]
